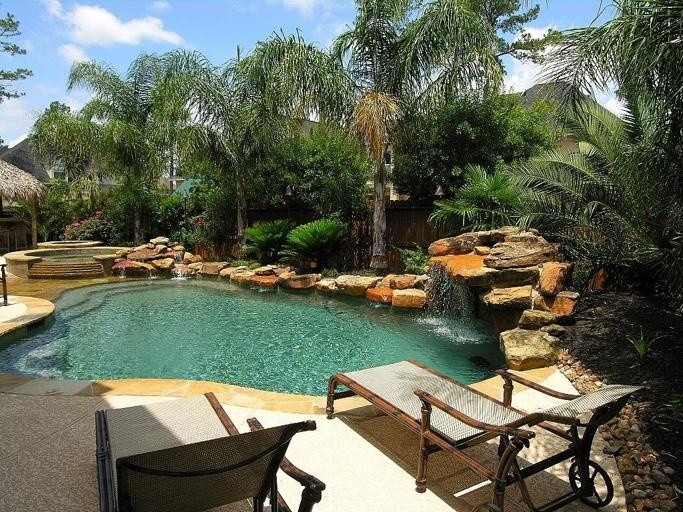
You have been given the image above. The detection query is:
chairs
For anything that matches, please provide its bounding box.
[325,357,646,512]
[93,392,325,511]
